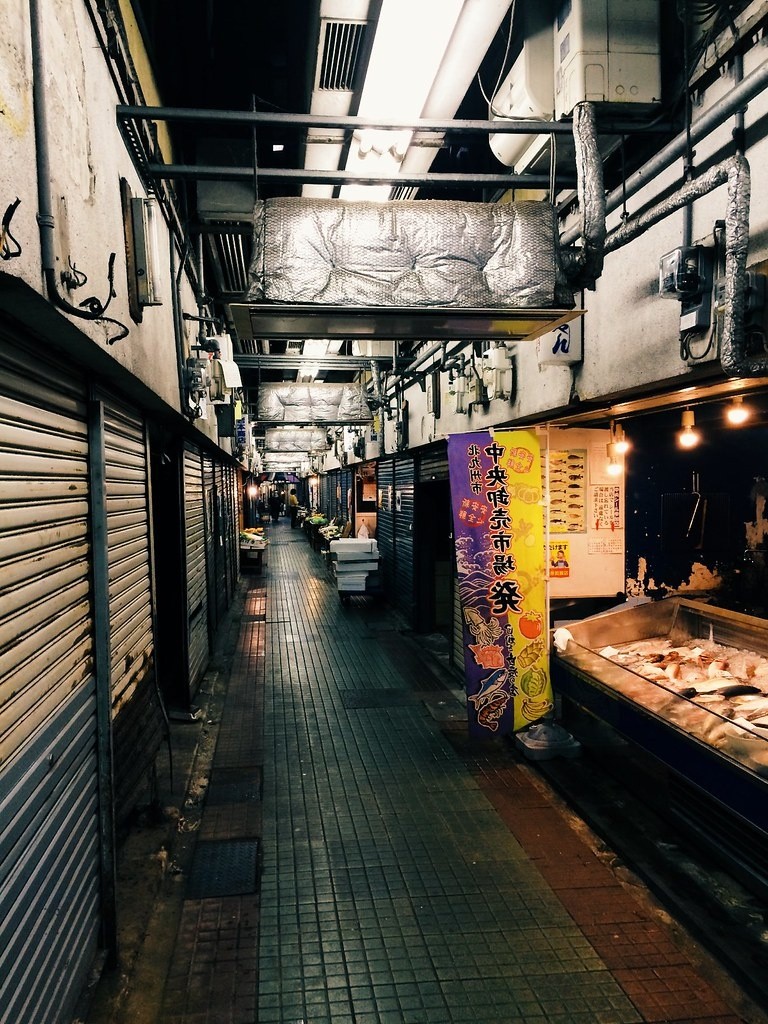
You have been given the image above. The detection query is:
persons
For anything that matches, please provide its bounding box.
[289,489,299,529]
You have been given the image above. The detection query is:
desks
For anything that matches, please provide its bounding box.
[241,545,266,574]
[302,516,342,570]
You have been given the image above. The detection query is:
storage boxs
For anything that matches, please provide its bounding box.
[329,537,379,590]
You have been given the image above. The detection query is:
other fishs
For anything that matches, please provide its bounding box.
[540,455,583,531]
[570,635,768,748]
